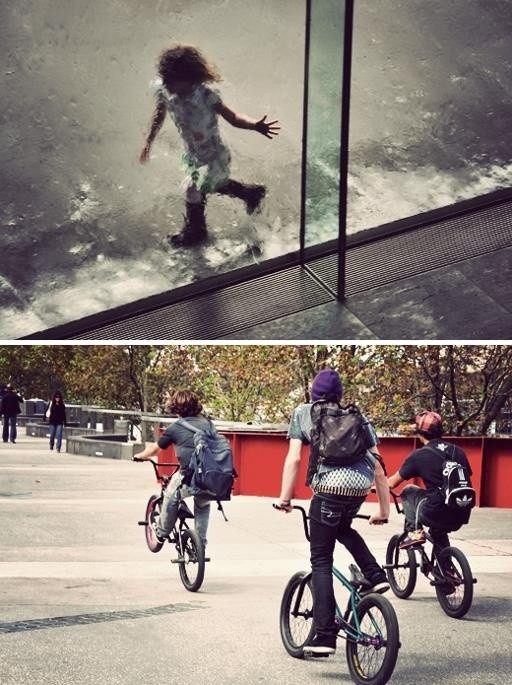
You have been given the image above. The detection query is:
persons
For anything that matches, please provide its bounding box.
[370,411,476,593]
[134,389,219,557]
[0,384,24,444]
[45,391,67,453]
[138,46,281,247]
[278,367,391,654]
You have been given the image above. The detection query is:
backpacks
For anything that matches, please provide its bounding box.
[175,418,234,500]
[422,443,476,510]
[310,399,374,465]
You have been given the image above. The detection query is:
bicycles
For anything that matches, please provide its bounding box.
[132,456,210,592]
[272,502,401,685]
[370,488,477,619]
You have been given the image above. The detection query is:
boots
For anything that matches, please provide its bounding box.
[218,179,265,215]
[167,201,208,246]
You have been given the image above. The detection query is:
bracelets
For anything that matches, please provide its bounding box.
[279,499,290,507]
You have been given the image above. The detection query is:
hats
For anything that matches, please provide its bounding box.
[311,370,342,404]
[412,412,444,436]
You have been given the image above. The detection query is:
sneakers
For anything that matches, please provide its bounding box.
[358,572,390,596]
[303,635,336,654]
[148,511,167,544]
[398,530,425,549]
